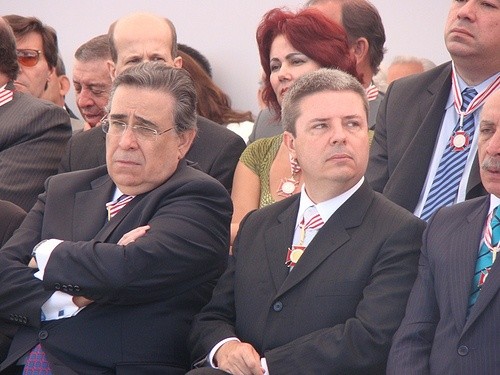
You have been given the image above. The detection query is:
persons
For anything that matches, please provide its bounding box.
[0,14,248,375]
[364,0,500,375]
[188,0,440,375]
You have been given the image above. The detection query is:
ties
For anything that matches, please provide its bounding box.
[467,206,500,318]
[420,88,473,223]
[104,194,128,223]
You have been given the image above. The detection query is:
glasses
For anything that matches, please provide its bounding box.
[15,48,45,67]
[99,114,176,144]
